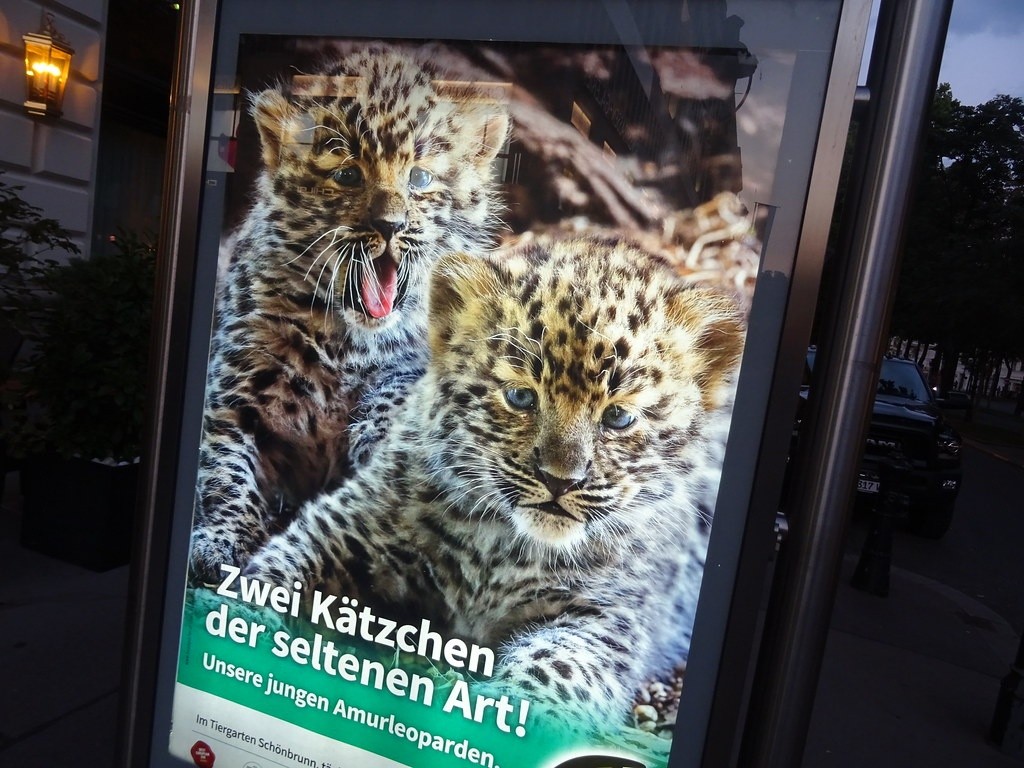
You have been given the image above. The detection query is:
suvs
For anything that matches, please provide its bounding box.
[784,351,972,538]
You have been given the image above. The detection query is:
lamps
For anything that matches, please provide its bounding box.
[21,13,76,122]
